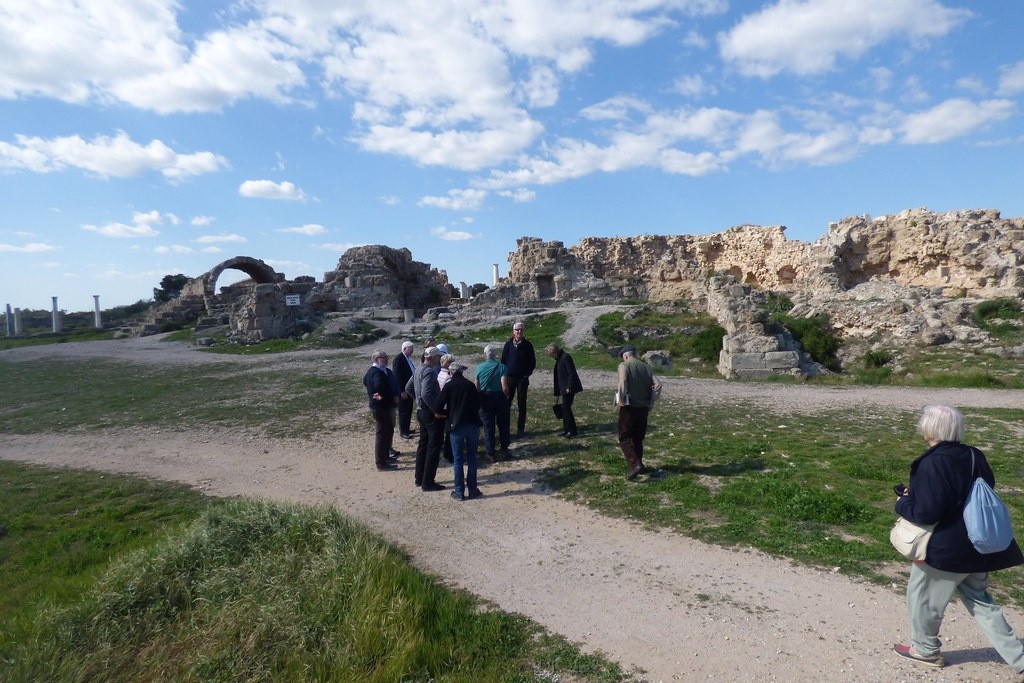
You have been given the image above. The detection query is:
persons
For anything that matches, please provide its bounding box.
[616,345,662,481]
[892,402,1024,683]
[363,349,399,471]
[405,346,446,491]
[475,344,512,463]
[389,341,466,463]
[547,342,583,438]
[440,362,483,501]
[501,323,536,437]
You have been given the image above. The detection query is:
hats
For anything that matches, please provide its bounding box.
[436,344,447,353]
[424,347,443,358]
[620,345,636,358]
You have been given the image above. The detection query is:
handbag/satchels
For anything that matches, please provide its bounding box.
[963,476,1015,554]
[553,403,563,419]
[889,515,936,564]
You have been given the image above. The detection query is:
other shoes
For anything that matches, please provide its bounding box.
[414,478,422,486]
[389,447,400,457]
[408,429,416,434]
[501,451,512,458]
[485,453,496,462]
[558,430,566,436]
[422,483,445,492]
[628,465,643,480]
[386,457,397,463]
[450,491,464,501]
[378,464,398,471]
[564,431,578,439]
[893,643,944,668]
[400,433,413,439]
[469,488,483,499]
